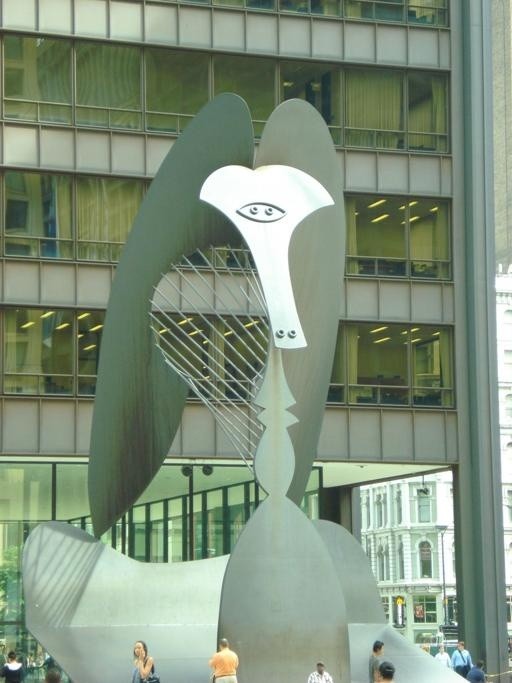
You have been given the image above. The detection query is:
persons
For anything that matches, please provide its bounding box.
[467,659,486,683]
[370,639,385,683]
[434,644,451,671]
[131,641,154,682]
[451,641,472,676]
[41,655,61,683]
[376,661,396,683]
[308,662,332,683]
[209,639,239,683]
[0,652,23,682]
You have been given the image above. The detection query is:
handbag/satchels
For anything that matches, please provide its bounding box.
[140,659,160,683]
